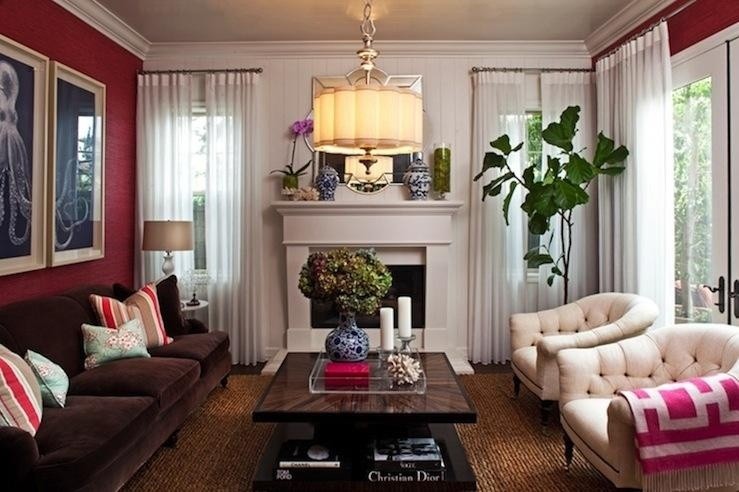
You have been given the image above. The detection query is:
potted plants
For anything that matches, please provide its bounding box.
[298,247,392,362]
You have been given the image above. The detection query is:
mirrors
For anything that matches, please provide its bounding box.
[310,66,423,186]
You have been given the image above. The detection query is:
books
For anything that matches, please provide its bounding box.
[273,438,344,481]
[365,436,447,483]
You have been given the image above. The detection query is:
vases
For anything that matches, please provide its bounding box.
[282,175,299,195]
[402,151,432,200]
[325,309,369,362]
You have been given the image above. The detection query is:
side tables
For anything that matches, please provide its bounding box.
[178,300,210,324]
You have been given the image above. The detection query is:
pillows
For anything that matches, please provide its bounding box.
[90,281,174,348]
[24,348,69,408]
[112,275,183,334]
[0,342,43,438]
[81,319,151,369]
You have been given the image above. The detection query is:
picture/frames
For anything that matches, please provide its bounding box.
[49,58,106,268]
[1,33,49,274]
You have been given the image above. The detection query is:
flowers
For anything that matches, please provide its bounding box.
[269,119,315,176]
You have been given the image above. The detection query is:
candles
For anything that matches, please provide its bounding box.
[380,307,394,351]
[398,297,412,337]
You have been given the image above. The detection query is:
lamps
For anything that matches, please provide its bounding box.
[312,7,423,174]
[344,155,393,183]
[142,221,194,274]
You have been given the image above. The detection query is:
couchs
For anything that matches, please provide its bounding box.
[0,284,233,492]
[510,292,659,424]
[555,322,739,492]
